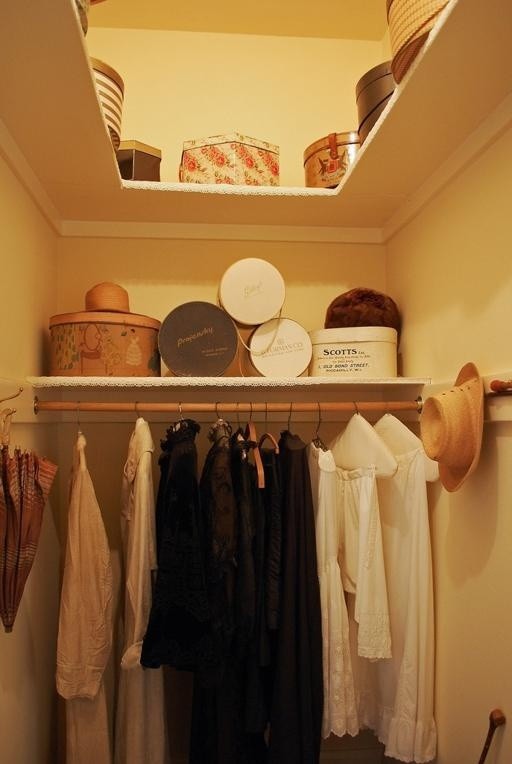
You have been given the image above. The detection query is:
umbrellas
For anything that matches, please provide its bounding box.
[0,410,59,633]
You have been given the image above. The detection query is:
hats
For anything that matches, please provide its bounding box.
[419,362,485,493]
